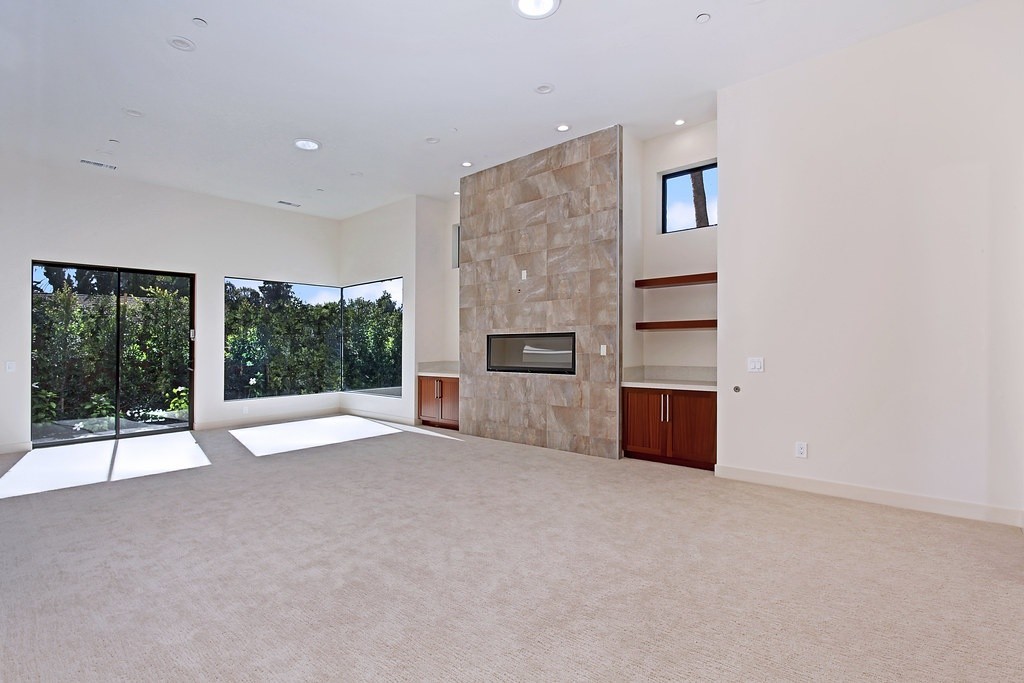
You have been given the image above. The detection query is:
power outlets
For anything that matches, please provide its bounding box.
[795,442,808,459]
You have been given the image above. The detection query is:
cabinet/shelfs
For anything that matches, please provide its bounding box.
[418,376,459,431]
[635,272,717,330]
[621,387,718,471]
